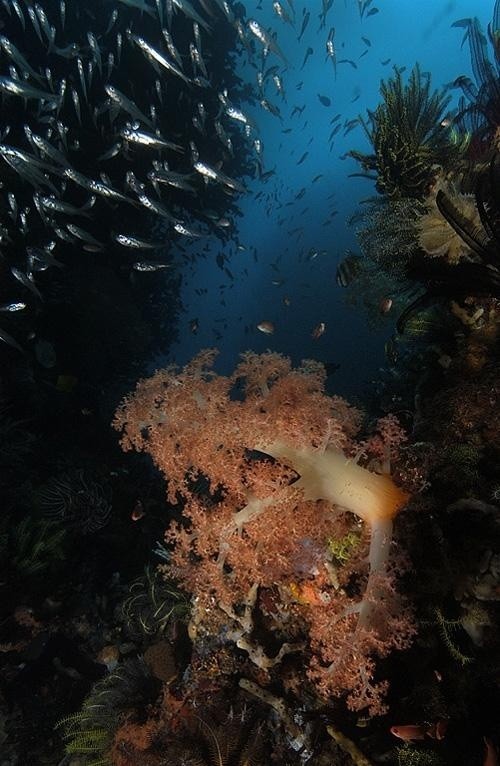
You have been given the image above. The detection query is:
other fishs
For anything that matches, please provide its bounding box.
[0,0,500,341]
